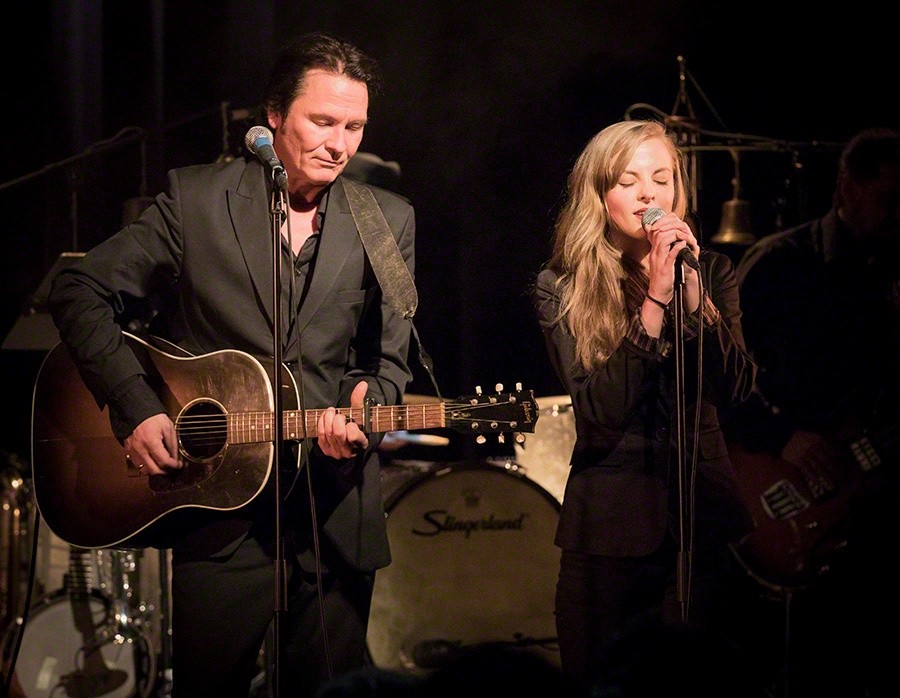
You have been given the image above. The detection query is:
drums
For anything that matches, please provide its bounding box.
[366,459,565,674]
[13,588,155,698]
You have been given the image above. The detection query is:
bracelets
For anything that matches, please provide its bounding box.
[645,290,669,311]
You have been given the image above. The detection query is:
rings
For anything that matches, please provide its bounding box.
[811,461,819,467]
[136,463,144,469]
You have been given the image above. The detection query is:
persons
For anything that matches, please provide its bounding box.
[534,119,766,698]
[736,123,900,698]
[48,33,416,697]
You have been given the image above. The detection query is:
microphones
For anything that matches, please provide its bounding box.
[245,126,288,185]
[643,205,700,270]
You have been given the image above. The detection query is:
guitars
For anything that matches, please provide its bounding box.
[29,324,540,551]
